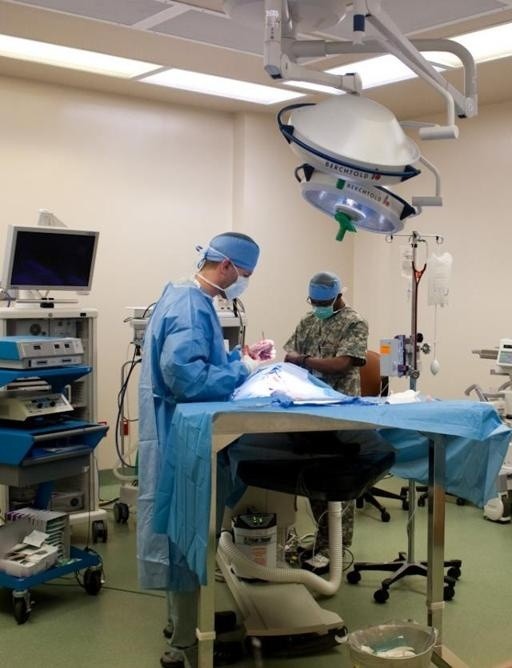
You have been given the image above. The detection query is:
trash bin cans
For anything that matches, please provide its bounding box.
[334,624,439,668]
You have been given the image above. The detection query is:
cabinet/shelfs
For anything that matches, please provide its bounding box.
[1,308,110,534]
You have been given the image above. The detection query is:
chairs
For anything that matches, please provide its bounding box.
[358,349,407,522]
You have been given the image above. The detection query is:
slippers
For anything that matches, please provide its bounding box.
[160,640,244,667]
[163,610,237,638]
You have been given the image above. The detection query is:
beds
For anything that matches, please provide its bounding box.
[151,399,512,667]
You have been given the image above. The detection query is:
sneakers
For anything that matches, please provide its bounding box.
[301,553,330,575]
[297,540,318,558]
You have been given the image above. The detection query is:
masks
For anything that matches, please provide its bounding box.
[219,258,250,301]
[311,293,340,320]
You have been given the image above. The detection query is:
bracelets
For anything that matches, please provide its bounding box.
[302,354,311,365]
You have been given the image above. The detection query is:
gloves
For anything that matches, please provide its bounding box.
[243,338,276,359]
[241,345,273,373]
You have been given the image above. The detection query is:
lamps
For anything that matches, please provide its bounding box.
[256,1,481,245]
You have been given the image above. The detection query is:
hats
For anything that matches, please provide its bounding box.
[205,231,260,273]
[309,272,340,301]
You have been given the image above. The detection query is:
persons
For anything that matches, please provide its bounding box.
[136,231,262,668]
[280,268,370,572]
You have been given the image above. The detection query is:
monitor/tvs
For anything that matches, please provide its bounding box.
[0,225,100,295]
